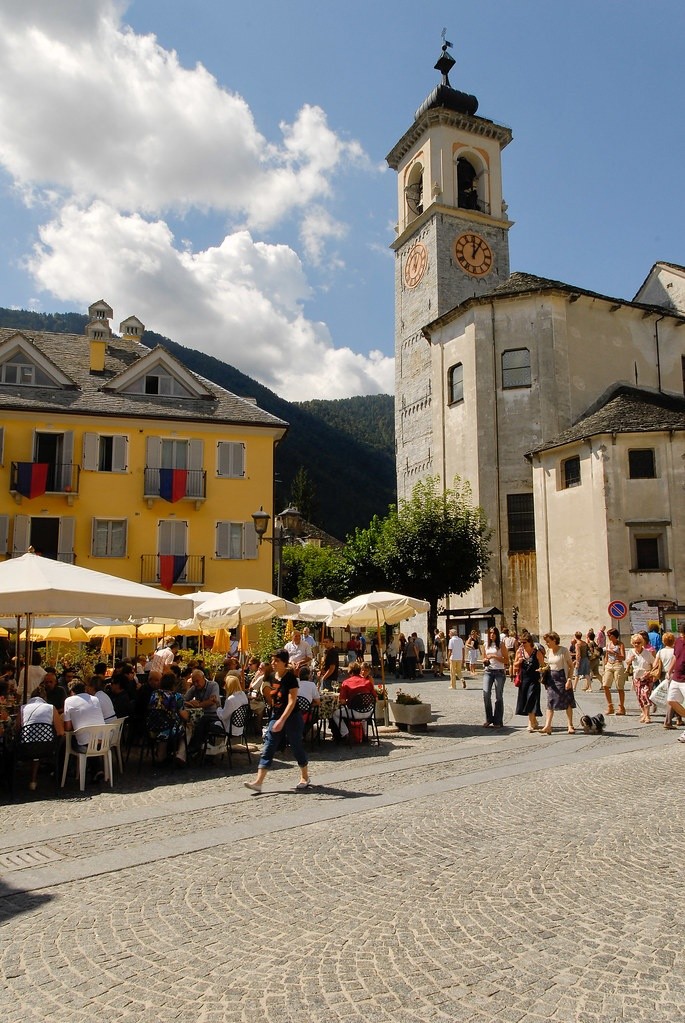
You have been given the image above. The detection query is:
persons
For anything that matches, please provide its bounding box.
[244,649,311,793]
[500,627,546,683]
[570,620,685,743]
[536,631,576,736]
[387,632,425,680]
[514,632,544,732]
[481,626,509,728]
[370,631,382,678]
[466,630,482,675]
[284,626,378,743]
[448,629,466,690]
[433,628,450,678]
[347,633,366,663]
[0,641,269,789]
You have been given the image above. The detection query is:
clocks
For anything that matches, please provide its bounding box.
[452,232,493,278]
[404,243,429,288]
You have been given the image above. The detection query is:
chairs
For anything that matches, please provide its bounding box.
[0,668,380,791]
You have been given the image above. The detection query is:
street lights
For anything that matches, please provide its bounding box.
[251,502,301,640]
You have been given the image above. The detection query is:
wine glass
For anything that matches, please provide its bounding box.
[331,681,339,694]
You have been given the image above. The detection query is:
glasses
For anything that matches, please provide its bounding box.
[633,642,641,644]
[521,641,529,644]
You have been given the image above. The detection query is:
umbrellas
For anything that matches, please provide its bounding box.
[279,597,344,647]
[0,546,301,705]
[326,591,430,700]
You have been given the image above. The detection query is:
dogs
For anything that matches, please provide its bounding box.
[580,713,607,735]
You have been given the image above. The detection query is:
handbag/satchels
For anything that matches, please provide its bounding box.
[514,650,522,687]
[648,678,669,711]
[650,651,661,677]
[589,643,603,661]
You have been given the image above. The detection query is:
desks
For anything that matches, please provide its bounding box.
[176,707,204,763]
[317,691,340,743]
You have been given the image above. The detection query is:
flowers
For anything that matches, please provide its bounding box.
[375,684,388,700]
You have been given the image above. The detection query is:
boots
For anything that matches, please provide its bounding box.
[605,703,614,714]
[616,705,625,714]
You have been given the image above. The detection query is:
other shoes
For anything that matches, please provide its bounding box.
[297,778,311,789]
[495,724,500,728]
[449,687,456,690]
[640,716,651,723]
[462,681,466,688]
[176,758,186,768]
[483,722,491,727]
[663,724,677,729]
[677,732,685,743]
[92,771,104,782]
[244,782,262,791]
[568,726,575,734]
[573,685,604,693]
[539,728,552,734]
[527,723,537,731]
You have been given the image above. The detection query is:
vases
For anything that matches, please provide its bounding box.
[371,698,393,726]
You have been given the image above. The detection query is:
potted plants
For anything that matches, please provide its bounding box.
[389,688,431,732]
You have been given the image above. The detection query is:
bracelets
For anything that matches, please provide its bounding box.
[568,678,571,681]
